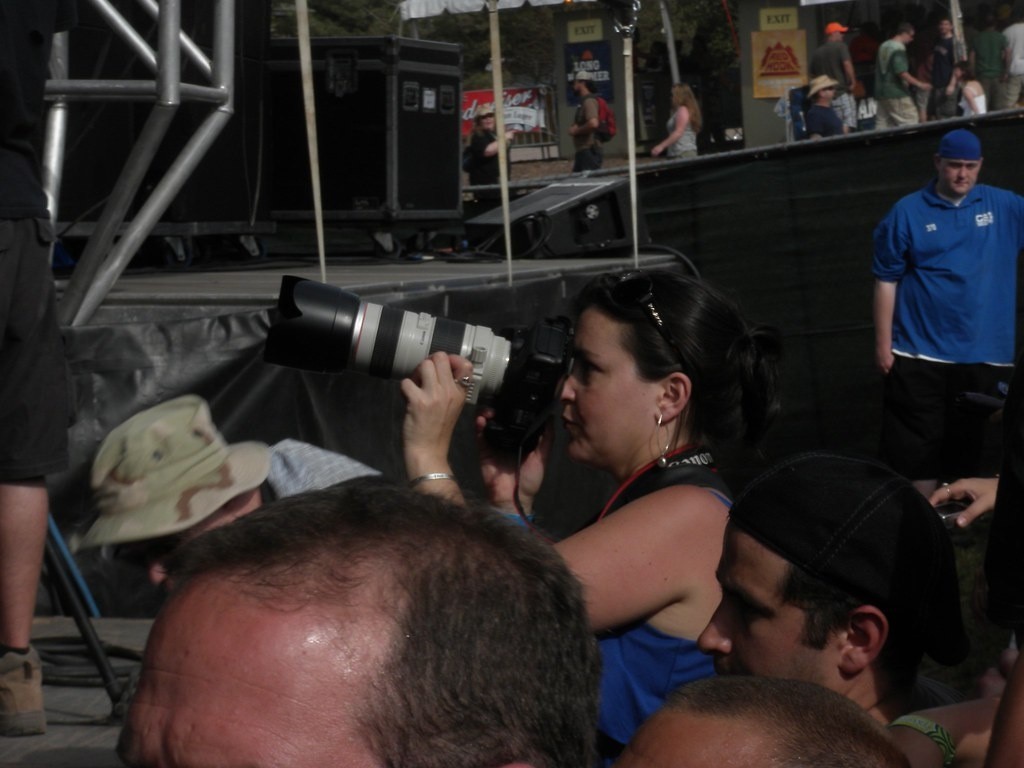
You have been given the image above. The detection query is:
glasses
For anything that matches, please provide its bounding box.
[615,269,686,374]
[476,112,495,120]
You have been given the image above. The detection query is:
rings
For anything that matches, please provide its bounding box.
[456,376,471,388]
[941,482,953,497]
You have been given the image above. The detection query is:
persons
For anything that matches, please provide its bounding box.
[0,0,84,736]
[466,103,518,207]
[819,22,860,134]
[864,132,1024,373]
[803,75,847,140]
[651,81,704,159]
[569,72,604,172]
[77,266,1024,768]
[874,22,933,131]
[914,9,1024,121]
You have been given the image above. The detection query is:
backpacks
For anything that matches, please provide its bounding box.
[582,96,616,142]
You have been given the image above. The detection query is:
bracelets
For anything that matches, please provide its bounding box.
[406,471,458,486]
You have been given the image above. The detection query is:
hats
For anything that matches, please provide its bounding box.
[826,23,848,33]
[937,129,982,161]
[77,395,273,546]
[574,70,590,81]
[730,452,967,666]
[805,75,839,98]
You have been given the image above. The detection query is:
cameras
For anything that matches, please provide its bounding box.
[263,274,576,463]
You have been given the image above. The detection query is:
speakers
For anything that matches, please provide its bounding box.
[465,177,651,257]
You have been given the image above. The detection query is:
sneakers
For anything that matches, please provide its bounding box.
[0,643,45,731]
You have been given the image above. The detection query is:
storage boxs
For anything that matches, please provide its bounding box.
[265,38,464,221]
